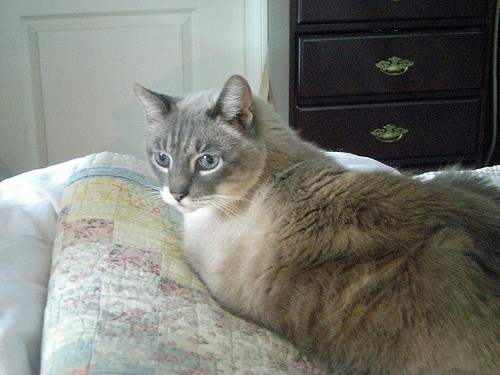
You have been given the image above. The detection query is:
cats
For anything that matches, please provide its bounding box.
[132,74,500,375]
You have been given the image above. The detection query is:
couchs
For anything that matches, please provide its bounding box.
[0,151,500,375]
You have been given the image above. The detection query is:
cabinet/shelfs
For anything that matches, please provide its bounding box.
[287,0,498,171]
